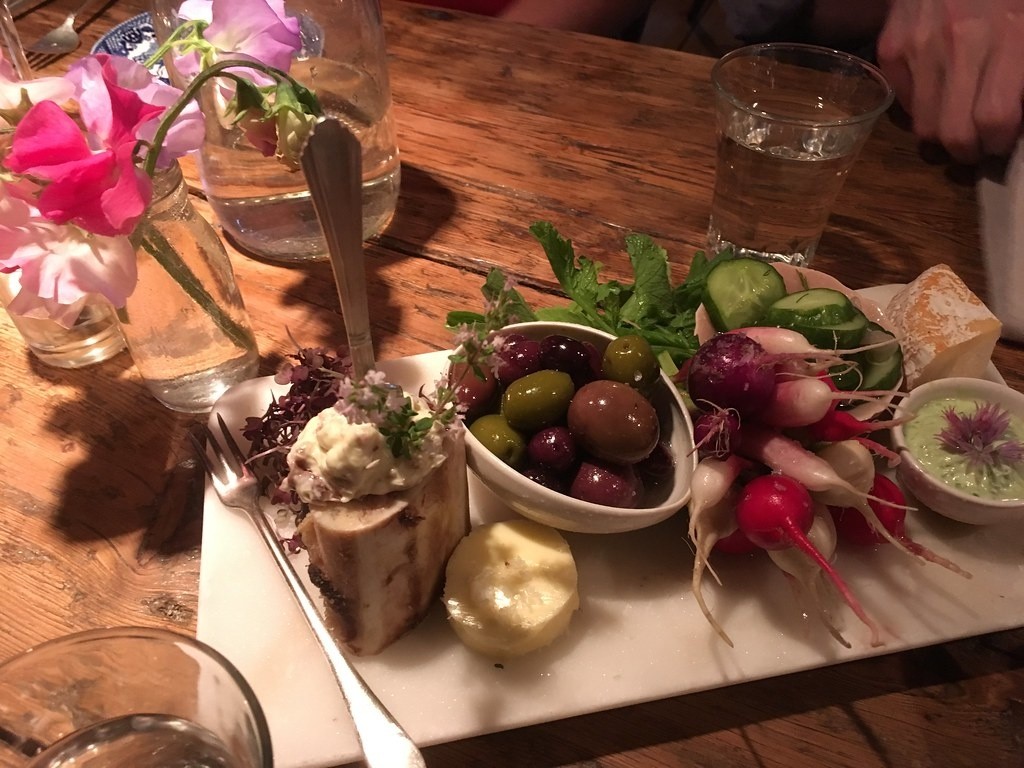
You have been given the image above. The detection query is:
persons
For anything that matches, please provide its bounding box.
[876,0,1023,164]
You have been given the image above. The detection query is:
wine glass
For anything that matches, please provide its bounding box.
[0,0,86,170]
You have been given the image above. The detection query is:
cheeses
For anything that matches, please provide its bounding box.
[883,262,1004,392]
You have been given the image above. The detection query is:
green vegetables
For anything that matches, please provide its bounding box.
[442,219,733,366]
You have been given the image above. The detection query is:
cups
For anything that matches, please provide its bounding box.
[196,1,400,260]
[152,0,188,92]
[0,209,125,368]
[705,42,895,265]
[0,627,274,767]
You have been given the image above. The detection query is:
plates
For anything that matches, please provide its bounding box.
[90,12,170,89]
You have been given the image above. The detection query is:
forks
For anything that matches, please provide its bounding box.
[20,0,97,54]
[188,413,427,768]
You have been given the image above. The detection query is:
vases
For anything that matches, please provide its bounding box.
[0,270,123,369]
[96,162,261,415]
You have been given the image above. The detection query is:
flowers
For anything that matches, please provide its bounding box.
[0,0,326,329]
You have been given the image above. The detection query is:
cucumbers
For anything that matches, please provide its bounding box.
[701,257,903,408]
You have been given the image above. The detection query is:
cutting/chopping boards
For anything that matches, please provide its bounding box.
[197,284,1023,768]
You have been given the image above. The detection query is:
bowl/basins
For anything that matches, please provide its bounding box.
[890,378,1024,526]
[443,322,698,534]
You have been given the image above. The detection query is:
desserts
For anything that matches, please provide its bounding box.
[284,285,513,657]
[442,520,581,658]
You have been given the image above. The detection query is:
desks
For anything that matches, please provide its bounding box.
[0,0,1024,768]
